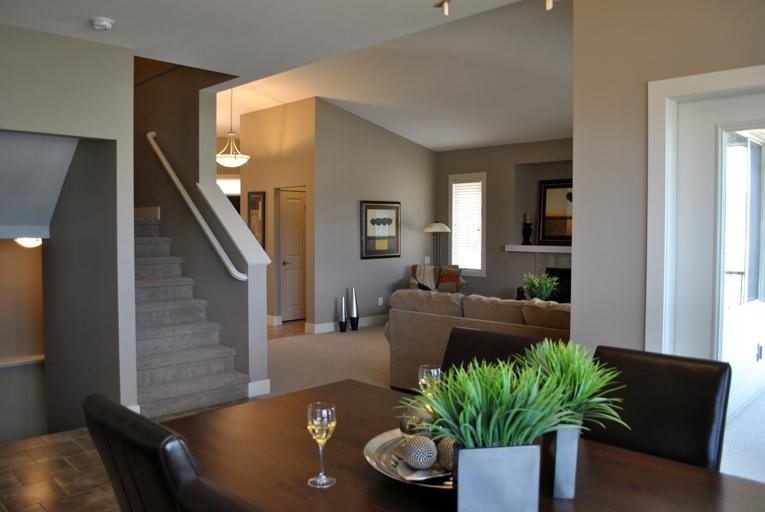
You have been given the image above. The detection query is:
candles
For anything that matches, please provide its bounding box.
[524,211,531,223]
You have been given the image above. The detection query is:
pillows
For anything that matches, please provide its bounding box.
[389,288,571,329]
[438,266,463,292]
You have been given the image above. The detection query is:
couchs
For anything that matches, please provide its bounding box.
[385,309,570,394]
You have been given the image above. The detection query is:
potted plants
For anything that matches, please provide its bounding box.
[391,357,591,512]
[511,336,631,499]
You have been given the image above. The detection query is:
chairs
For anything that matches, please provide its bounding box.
[440,327,531,392]
[408,264,469,293]
[546,267,571,303]
[85,394,203,511]
[581,346,731,472]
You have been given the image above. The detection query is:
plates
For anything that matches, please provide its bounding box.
[363,426,454,490]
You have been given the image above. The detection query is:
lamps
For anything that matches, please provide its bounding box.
[216,88,251,167]
[423,220,451,266]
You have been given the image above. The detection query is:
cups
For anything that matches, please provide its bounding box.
[418,364,441,397]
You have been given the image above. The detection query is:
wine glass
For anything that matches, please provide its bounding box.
[306,401,337,489]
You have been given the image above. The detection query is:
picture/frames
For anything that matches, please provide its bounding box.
[247,191,265,249]
[360,201,401,260]
[536,177,572,246]
